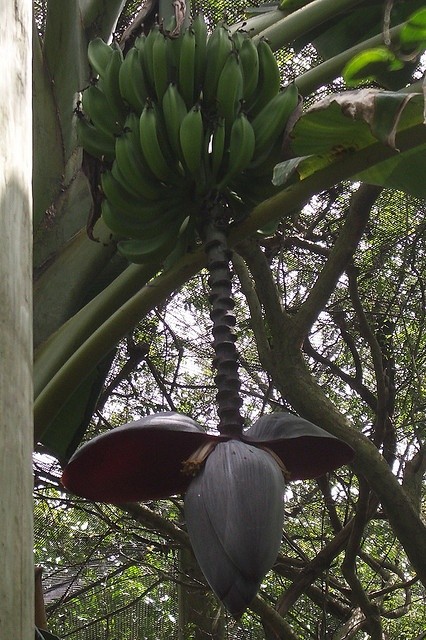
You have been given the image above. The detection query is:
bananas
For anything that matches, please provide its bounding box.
[74,12,301,266]
